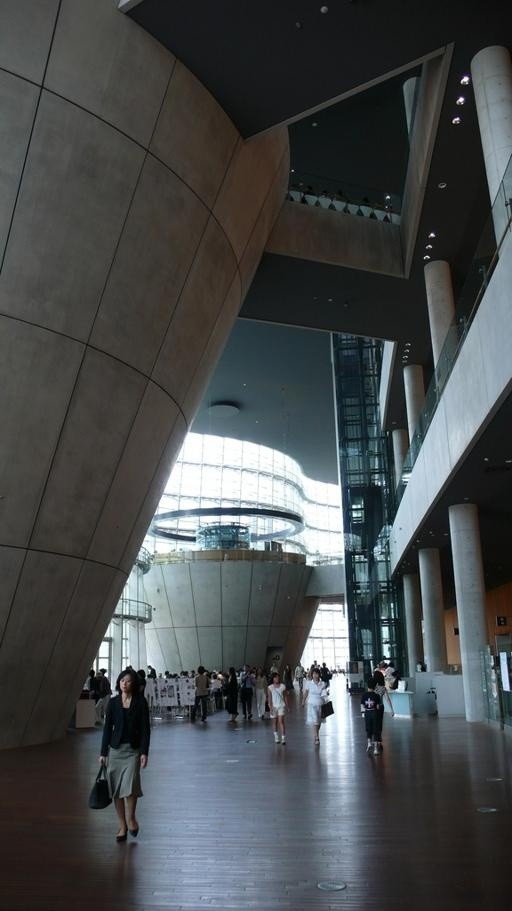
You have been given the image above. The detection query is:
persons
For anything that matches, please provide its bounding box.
[361,678,387,757]
[265,672,291,747]
[99,667,153,847]
[85,659,333,725]
[373,660,426,691]
[368,670,395,752]
[300,667,330,748]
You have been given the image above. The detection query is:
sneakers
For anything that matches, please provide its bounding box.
[366,744,383,755]
[315,739,320,745]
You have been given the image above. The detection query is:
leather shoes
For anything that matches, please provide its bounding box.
[116,826,127,841]
[129,826,138,837]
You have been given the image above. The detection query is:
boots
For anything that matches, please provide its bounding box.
[274,732,286,744]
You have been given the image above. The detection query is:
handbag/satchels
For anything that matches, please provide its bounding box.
[321,701,334,718]
[89,779,112,809]
